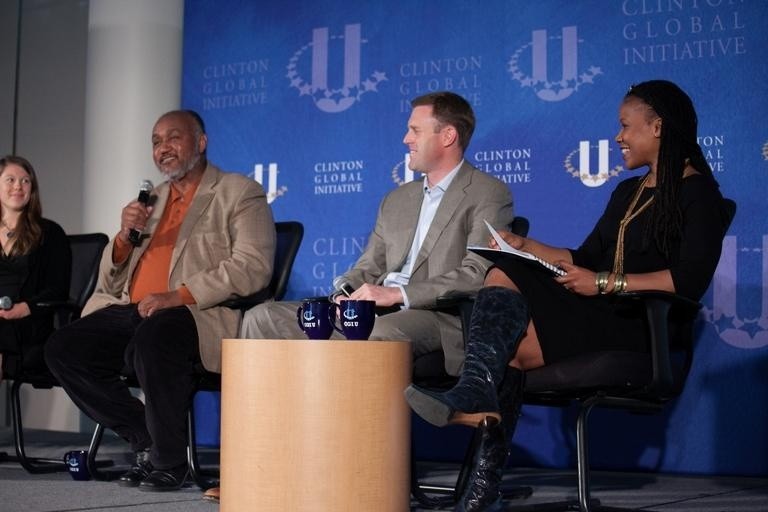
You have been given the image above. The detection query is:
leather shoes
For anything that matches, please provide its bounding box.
[116,447,152,487]
[203,488,220,501]
[137,460,191,493]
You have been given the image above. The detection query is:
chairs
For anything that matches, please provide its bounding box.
[86,221,304,490]
[455,198,736,512]
[0,232,115,474]
[301,217,530,509]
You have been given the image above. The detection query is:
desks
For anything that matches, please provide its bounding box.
[219,338,412,512]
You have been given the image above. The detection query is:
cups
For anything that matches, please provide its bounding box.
[297,301,336,340]
[328,300,376,340]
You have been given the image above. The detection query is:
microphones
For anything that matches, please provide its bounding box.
[332,275,355,298]
[128,180,154,246]
[0,295,11,310]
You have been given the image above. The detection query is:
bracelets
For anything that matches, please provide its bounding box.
[595,271,611,295]
[608,273,627,295]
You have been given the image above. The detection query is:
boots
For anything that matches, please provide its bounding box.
[452,365,526,511]
[403,284,532,439]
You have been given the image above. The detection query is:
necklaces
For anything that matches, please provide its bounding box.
[612,166,691,276]
[1,218,17,238]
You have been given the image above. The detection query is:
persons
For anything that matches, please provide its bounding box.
[43,110,276,492]
[1,155,75,390]
[201,92,514,505]
[404,80,727,510]
